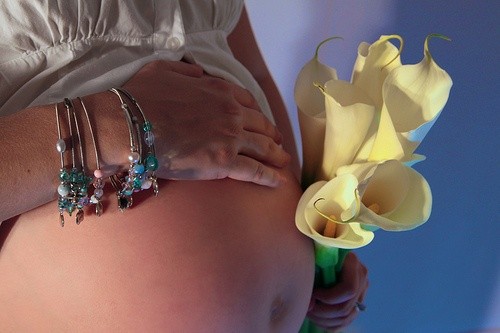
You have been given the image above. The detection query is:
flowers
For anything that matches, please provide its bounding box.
[293,31,453,333]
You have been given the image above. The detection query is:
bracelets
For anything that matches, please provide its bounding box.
[54,82,158,227]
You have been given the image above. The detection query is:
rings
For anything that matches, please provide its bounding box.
[355,300,367,312]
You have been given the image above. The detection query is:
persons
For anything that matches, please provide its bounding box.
[0,0,371,332]
